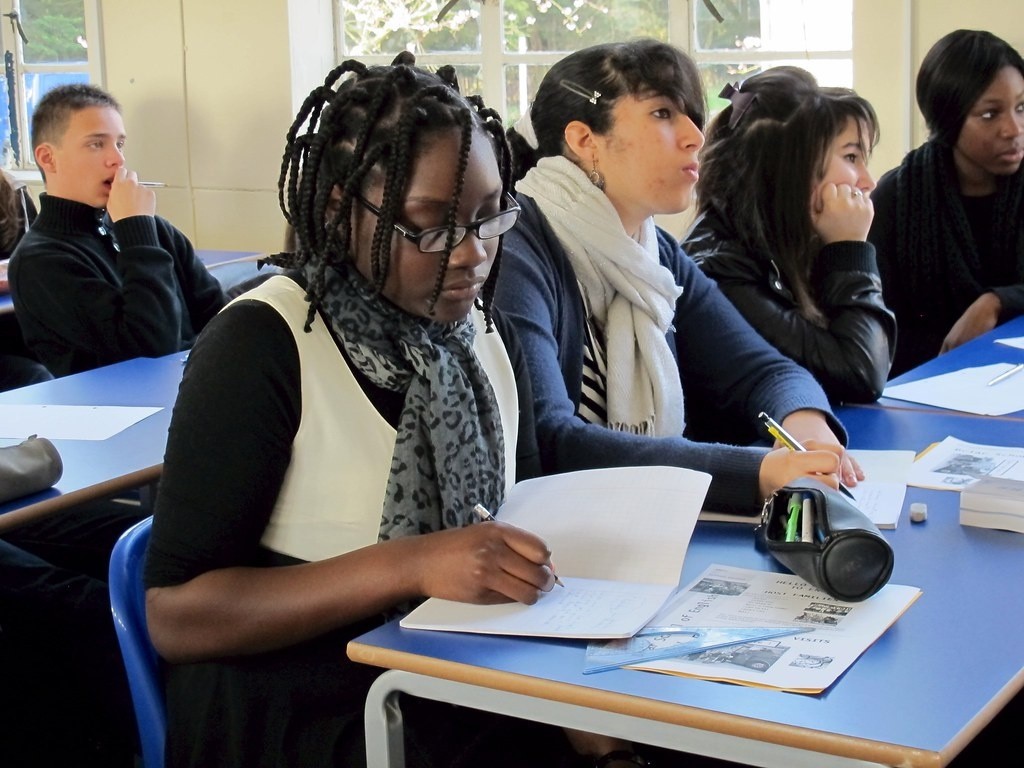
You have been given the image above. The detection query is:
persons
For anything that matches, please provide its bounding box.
[867,30,1024,367]
[679,66,896,446]
[143,52,651,768]
[481,36,867,516]
[0,83,234,768]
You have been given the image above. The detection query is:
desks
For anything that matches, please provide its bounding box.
[345,314,1024,768]
[0,347,193,569]
[0,250,265,313]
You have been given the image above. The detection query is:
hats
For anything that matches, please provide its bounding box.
[915,29,1023,153]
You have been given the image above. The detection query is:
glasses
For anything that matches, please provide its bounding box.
[355,194,522,253]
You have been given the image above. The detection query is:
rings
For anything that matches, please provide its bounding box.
[852,192,862,197]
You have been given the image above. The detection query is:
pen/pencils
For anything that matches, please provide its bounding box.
[988,363,1024,387]
[757,410,859,502]
[138,181,169,190]
[777,491,828,545]
[472,501,569,590]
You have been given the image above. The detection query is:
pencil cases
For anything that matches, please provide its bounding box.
[761,476,895,604]
[0,432,64,505]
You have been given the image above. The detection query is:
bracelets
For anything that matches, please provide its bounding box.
[596,749,650,768]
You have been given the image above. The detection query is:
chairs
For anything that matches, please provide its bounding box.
[206,262,284,292]
[108,513,165,768]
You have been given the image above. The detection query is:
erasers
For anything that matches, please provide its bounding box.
[910,502,929,523]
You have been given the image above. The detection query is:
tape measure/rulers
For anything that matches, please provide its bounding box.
[580,625,818,676]
